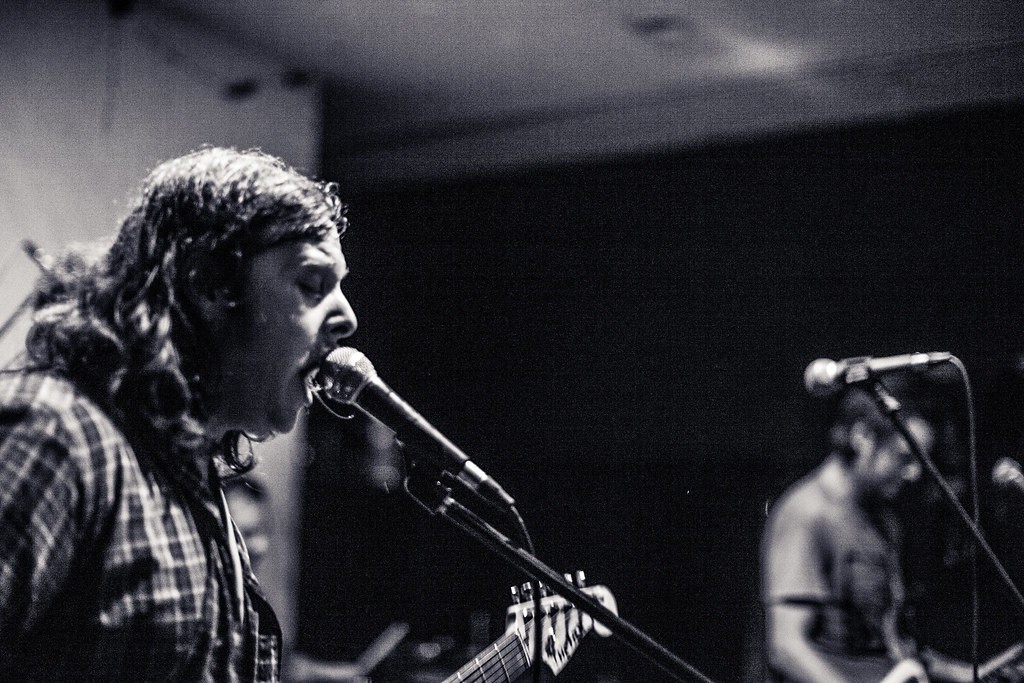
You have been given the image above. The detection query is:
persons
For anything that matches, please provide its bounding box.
[0,146,356,683]
[760,368,975,683]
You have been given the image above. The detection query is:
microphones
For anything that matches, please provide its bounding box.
[804,354,953,398]
[322,346,516,512]
[993,458,1024,494]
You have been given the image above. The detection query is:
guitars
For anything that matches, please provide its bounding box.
[437,569,620,683]
